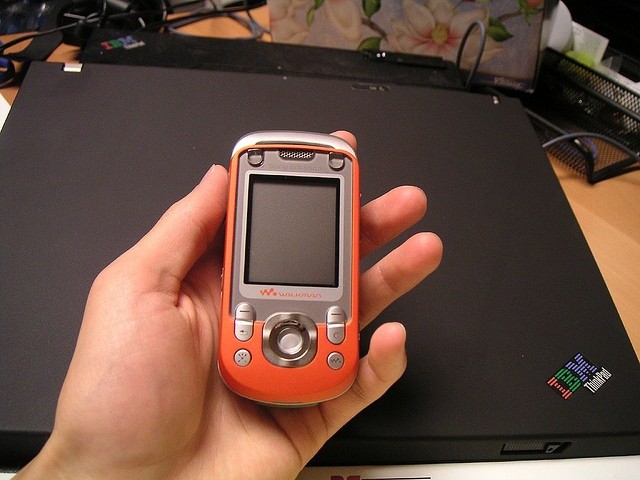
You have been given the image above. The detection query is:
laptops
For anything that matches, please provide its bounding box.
[0,58,639,454]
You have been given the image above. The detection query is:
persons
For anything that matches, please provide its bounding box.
[12,129,445,479]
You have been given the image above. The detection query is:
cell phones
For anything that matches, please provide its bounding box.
[215,128,362,411]
